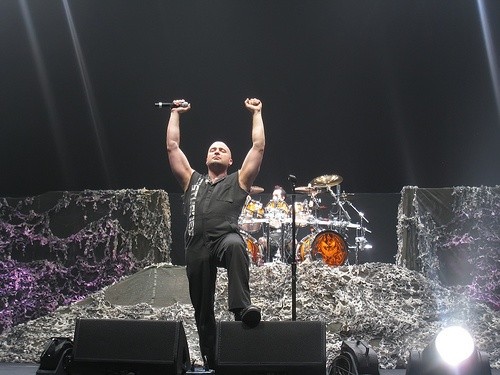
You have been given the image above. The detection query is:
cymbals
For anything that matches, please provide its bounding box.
[250,186,264,195]
[308,175,343,188]
[292,186,318,194]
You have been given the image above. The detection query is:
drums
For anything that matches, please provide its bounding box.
[264,220,291,247]
[240,230,262,267]
[265,199,289,218]
[245,200,264,217]
[243,218,262,234]
[296,230,348,267]
[290,201,309,216]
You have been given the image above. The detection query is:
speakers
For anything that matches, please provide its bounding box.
[70,316,191,375]
[214,319,326,375]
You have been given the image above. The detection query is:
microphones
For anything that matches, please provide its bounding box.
[155,101,188,107]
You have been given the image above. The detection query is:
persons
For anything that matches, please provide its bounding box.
[167,99,265,370]
[258,185,297,262]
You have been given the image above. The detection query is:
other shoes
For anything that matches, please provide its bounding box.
[235,305,261,322]
